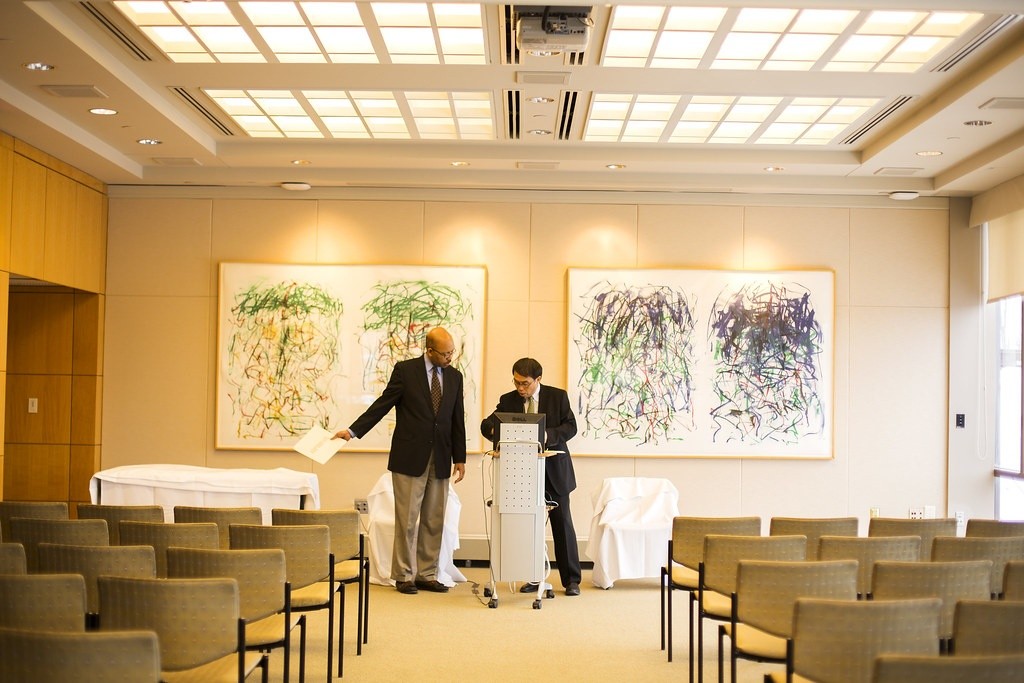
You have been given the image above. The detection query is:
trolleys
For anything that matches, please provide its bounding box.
[485,441,556,609]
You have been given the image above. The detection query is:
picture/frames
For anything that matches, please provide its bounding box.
[563,265,838,461]
[216,259,489,455]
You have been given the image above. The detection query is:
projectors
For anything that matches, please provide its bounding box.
[516,16,590,52]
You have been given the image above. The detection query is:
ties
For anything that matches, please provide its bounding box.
[430,366,442,420]
[527,396,535,414]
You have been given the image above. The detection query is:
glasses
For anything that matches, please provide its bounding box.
[512,379,535,388]
[429,347,456,358]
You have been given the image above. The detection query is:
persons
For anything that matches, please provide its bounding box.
[480,358,582,596]
[331,328,466,594]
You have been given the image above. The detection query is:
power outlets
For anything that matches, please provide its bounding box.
[353,500,368,514]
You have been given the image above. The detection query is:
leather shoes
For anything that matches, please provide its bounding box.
[396,580,417,594]
[520,582,538,593]
[414,578,449,591]
[566,582,580,595]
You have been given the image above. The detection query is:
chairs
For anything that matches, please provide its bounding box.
[660,516,1024,683]
[582,477,678,589]
[365,470,467,589]
[0,499,373,683]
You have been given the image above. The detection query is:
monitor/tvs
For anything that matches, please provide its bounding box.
[494,412,546,454]
